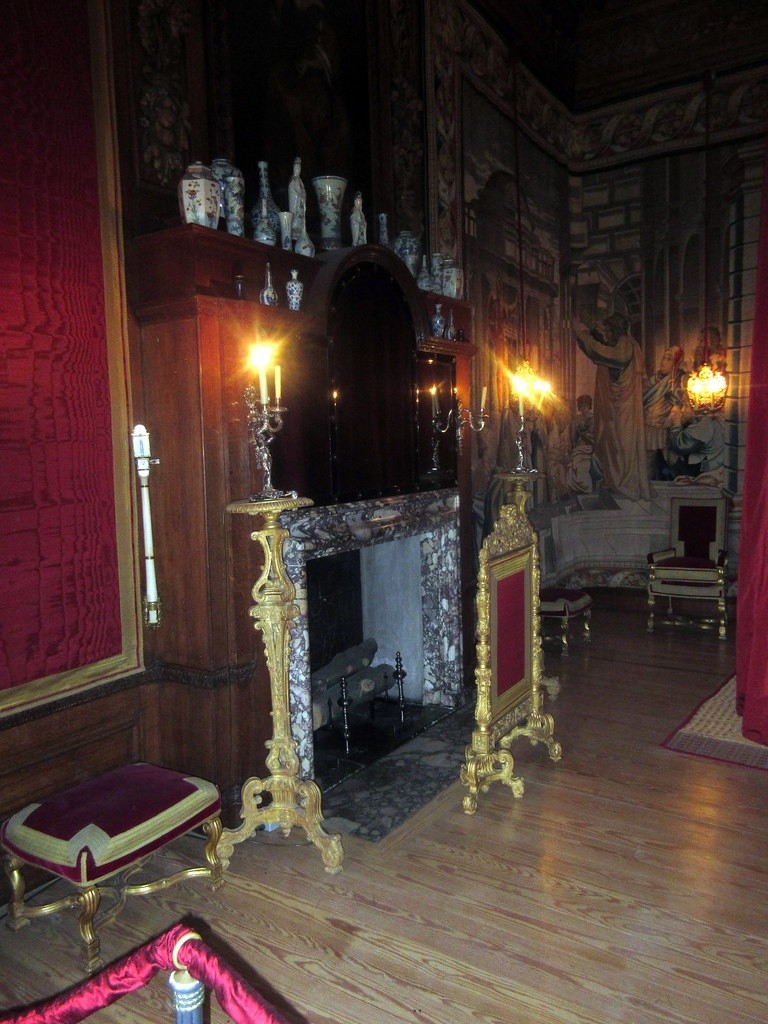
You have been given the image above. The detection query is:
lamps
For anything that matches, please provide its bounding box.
[684,72,729,416]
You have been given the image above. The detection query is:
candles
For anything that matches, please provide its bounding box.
[274,365,281,399]
[249,334,279,404]
[429,384,441,410]
[537,382,551,409]
[480,386,487,408]
[512,377,530,415]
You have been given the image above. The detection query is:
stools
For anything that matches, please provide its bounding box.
[0,763,225,974]
[537,588,594,646]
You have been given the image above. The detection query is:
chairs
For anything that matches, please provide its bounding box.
[646,497,733,626]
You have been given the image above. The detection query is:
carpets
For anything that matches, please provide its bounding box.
[654,670,767,772]
